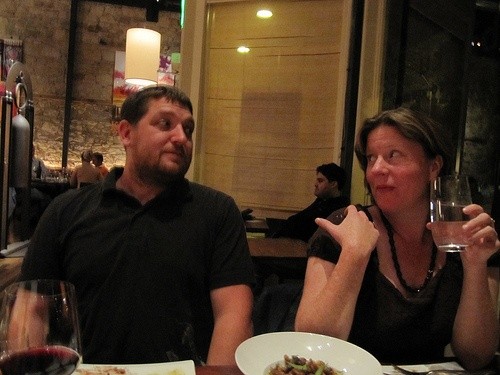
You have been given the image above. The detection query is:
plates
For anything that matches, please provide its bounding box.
[72,360,196,375]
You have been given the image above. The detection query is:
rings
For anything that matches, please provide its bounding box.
[491,218,493,223]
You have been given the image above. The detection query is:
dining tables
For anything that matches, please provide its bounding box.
[0,365,500,375]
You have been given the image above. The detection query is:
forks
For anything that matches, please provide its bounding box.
[391,362,468,375]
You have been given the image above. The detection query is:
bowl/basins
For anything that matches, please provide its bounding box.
[235,331,383,375]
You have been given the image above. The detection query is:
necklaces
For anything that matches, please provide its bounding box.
[381,211,438,297]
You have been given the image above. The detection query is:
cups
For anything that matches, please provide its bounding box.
[0,280,82,375]
[430,175,474,251]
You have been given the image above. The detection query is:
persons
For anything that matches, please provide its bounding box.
[294,104,500,371]
[70,151,103,190]
[7,86,259,366]
[92,152,109,176]
[267,163,340,239]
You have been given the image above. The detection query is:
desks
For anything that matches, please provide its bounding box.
[32,176,69,196]
[241,229,310,259]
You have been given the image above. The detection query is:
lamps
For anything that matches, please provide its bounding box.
[124,28,161,83]
